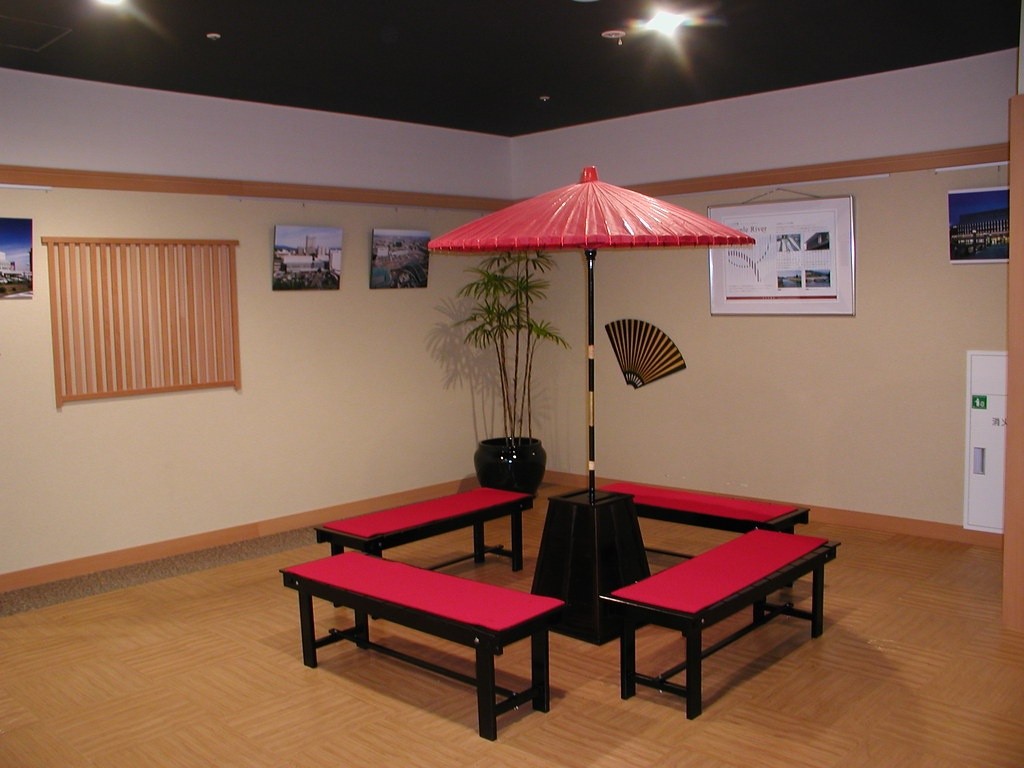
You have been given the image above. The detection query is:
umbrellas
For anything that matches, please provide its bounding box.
[429,167,756,502]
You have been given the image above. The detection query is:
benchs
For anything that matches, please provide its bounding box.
[597,529,842,722]
[312,487,538,575]
[280,552,566,741]
[595,480,812,560]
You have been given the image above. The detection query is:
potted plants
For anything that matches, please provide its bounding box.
[455,247,573,503]
